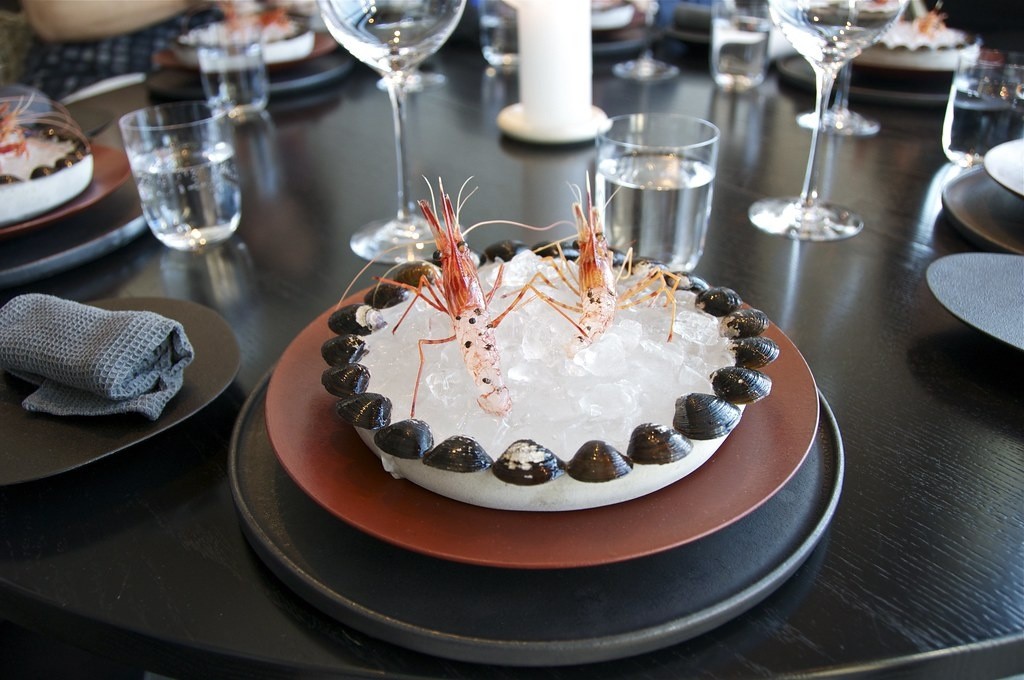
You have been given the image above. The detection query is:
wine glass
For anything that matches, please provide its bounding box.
[315,0,488,266]
[610,0,680,82]
[795,1,882,138]
[747,0,910,241]
[375,66,448,94]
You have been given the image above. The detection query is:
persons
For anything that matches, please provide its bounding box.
[21,0,317,42]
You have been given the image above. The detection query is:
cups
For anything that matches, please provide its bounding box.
[196,20,270,118]
[118,103,242,253]
[477,0,520,66]
[592,112,721,273]
[710,0,778,91]
[941,57,1024,167]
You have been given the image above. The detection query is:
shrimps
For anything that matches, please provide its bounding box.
[0,93,91,161]
[501,169,681,360]
[184,0,292,34]
[336,174,590,417]
[911,0,947,43]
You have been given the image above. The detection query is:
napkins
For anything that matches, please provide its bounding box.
[0,291,194,422]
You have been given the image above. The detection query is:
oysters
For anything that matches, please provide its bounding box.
[0,123,91,186]
[173,13,313,51]
[321,240,780,487]
[871,32,983,54]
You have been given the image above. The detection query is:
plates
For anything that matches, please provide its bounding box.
[925,252,1024,353]
[848,36,1007,79]
[1,115,130,239]
[1,296,244,488]
[150,19,337,74]
[266,279,820,571]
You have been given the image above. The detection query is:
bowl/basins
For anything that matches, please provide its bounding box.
[983,137,1024,201]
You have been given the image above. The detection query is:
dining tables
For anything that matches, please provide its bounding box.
[0,2,1024,680]
